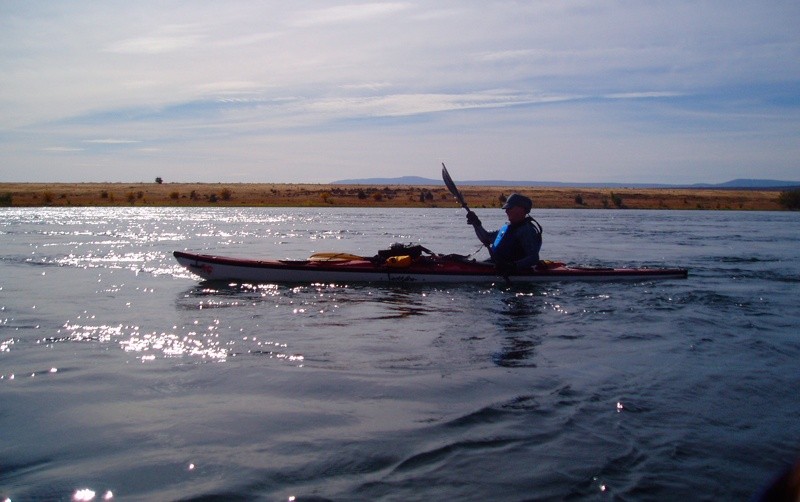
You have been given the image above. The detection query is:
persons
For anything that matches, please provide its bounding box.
[466,193,542,265]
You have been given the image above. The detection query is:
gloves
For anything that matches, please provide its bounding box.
[466,210,481,227]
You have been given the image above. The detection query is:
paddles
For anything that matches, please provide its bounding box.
[440,162,493,257]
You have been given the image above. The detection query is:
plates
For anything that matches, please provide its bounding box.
[278,260,312,265]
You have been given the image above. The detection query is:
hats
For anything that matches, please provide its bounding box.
[503,193,532,214]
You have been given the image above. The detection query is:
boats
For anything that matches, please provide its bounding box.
[173,251,689,286]
